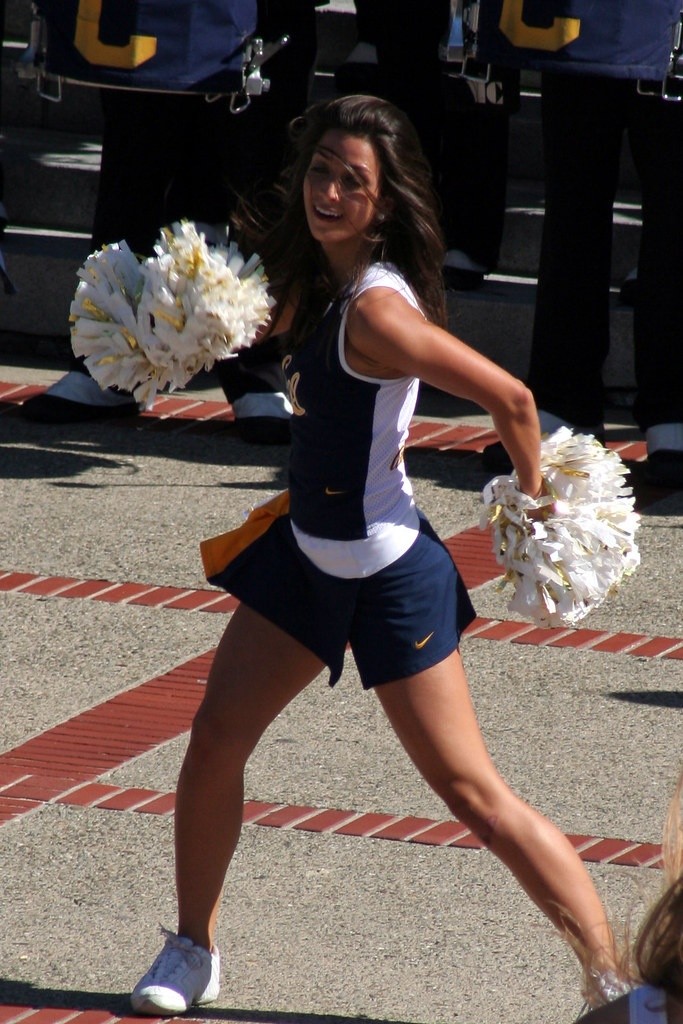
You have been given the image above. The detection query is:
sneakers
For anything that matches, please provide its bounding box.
[580,968,634,1010]
[129,924,221,1016]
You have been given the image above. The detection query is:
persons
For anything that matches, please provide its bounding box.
[573,766,682,1024]
[0,1,683,480]
[131,94,630,1015]
